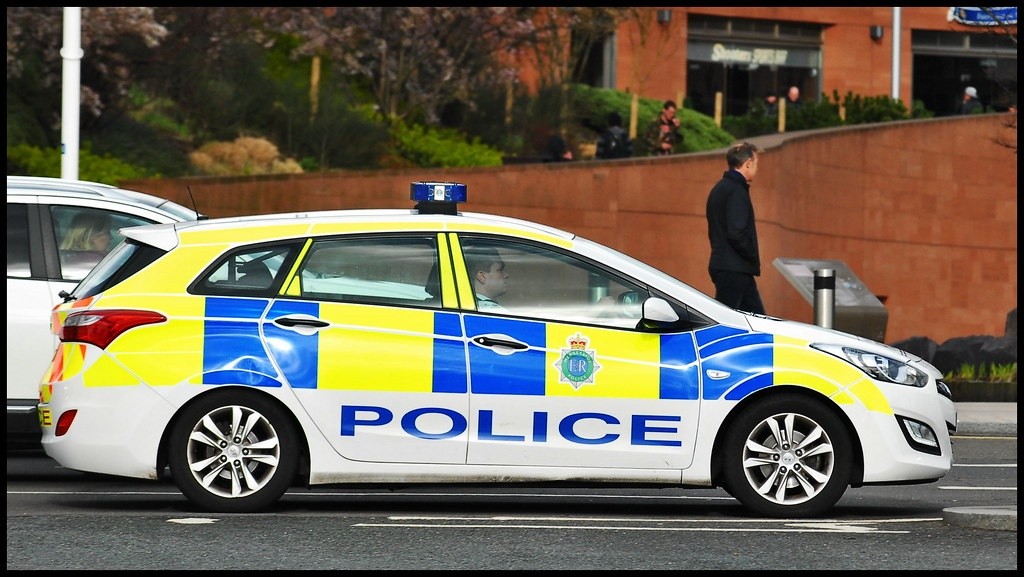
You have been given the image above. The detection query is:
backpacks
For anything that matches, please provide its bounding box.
[604,131,630,162]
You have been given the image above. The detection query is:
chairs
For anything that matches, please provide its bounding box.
[423,263,442,306]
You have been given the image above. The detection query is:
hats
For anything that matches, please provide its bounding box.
[965,87,977,98]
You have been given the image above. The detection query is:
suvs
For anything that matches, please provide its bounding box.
[6,175,431,412]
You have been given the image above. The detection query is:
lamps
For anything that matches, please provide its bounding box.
[870,24,885,40]
[658,9,672,25]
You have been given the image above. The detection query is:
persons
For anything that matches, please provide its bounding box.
[545,99,685,163]
[765,86,803,106]
[706,143,766,315]
[464,245,510,312]
[962,87,982,116]
[58,211,114,270]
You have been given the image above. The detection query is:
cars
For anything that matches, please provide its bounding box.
[37,178,958,517]
